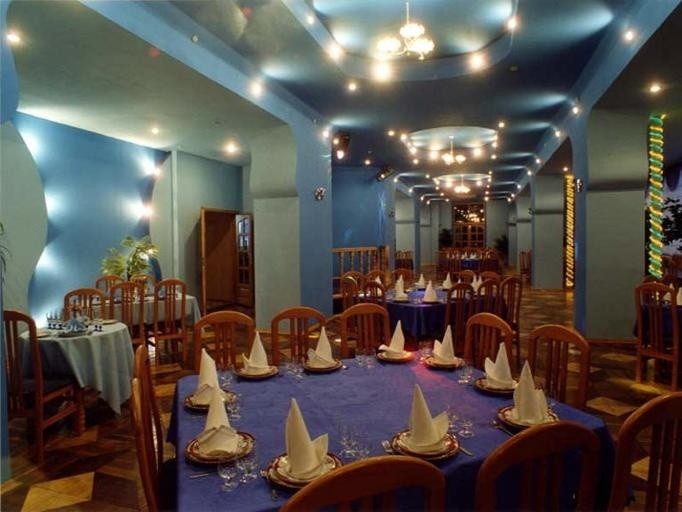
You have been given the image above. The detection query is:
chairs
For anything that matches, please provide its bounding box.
[609,392,682,511]
[97,276,124,292]
[130,273,155,282]
[131,378,162,511]
[194,311,256,375]
[365,270,387,287]
[478,271,499,282]
[272,307,326,365]
[477,280,500,315]
[520,250,533,278]
[279,454,446,512]
[146,279,188,364]
[397,250,415,268]
[436,246,500,269]
[445,283,475,356]
[341,276,360,312]
[110,282,145,345]
[528,326,588,409]
[500,276,523,351]
[340,303,389,359]
[391,269,411,287]
[134,344,164,475]
[364,281,387,309]
[633,284,678,403]
[18,320,135,416]
[342,271,365,291]
[473,421,600,512]
[459,270,477,285]
[64,288,106,319]
[4,309,86,464]
[464,313,513,374]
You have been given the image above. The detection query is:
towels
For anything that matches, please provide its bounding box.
[417,274,426,288]
[369,276,381,295]
[514,363,551,422]
[285,396,330,479]
[401,384,450,447]
[307,327,333,364]
[395,275,404,289]
[486,343,515,385]
[240,333,269,373]
[451,279,464,298]
[432,326,456,362]
[477,276,484,293]
[423,282,438,301]
[192,350,223,400]
[472,276,476,292]
[442,274,453,288]
[380,320,407,355]
[394,283,407,300]
[197,386,241,456]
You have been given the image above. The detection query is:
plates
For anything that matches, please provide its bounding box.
[392,429,460,461]
[377,352,414,363]
[267,451,343,490]
[302,359,342,371]
[234,365,279,379]
[425,356,465,368]
[497,405,559,430]
[184,431,254,464]
[184,389,238,410]
[475,376,522,395]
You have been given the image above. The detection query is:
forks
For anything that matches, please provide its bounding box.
[381,440,394,456]
[189,467,222,479]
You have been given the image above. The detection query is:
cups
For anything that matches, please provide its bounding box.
[418,341,433,363]
[217,452,238,494]
[458,418,476,438]
[285,358,295,374]
[356,439,372,462]
[454,358,474,385]
[366,349,377,368]
[235,441,259,483]
[446,411,459,429]
[293,356,306,380]
[223,367,233,387]
[339,426,358,457]
[354,349,366,368]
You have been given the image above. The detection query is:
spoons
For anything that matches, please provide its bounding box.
[488,419,515,437]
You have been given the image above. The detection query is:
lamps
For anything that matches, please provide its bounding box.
[441,140,466,165]
[373,0,435,59]
[455,175,470,193]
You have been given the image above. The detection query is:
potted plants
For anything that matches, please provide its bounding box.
[100,236,158,280]
